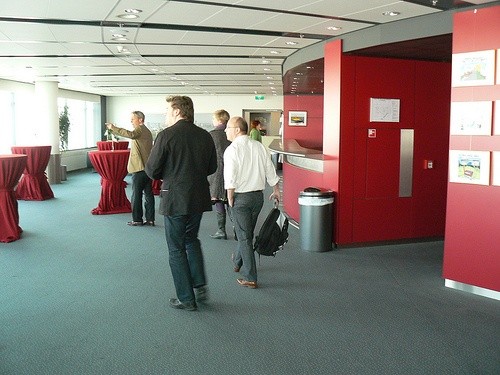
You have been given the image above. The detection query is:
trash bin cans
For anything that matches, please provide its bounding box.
[297,187,335,253]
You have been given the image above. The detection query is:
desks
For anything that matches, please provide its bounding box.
[87,150,132,215]
[0,154,28,243]
[96,141,130,151]
[9,145,54,201]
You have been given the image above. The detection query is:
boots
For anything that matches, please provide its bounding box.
[227,207,238,241]
[209,209,227,240]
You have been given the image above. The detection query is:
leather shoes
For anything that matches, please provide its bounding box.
[232,253,239,272]
[237,279,257,289]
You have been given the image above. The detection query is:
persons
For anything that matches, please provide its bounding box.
[105,111,155,227]
[210,110,238,240]
[224,117,281,288]
[250,120,262,143]
[144,96,217,310]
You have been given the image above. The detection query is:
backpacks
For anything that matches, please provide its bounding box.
[253,202,289,257]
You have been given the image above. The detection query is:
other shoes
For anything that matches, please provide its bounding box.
[143,220,154,226]
[127,220,143,226]
[169,298,197,311]
[194,284,206,288]
